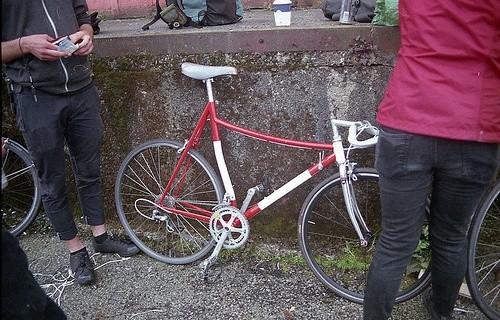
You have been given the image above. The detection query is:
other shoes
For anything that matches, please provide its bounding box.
[68,247,97,285]
[422,281,456,320]
[91,235,143,258]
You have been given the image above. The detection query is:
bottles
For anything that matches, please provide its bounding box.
[339,0,358,24]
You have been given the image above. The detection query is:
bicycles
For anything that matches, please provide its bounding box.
[465,178,500,320]
[114,61,433,306]
[0,135,42,238]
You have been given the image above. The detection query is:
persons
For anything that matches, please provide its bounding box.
[363,0,500,320]
[0,0,140,286]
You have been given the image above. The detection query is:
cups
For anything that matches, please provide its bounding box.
[274,0,292,26]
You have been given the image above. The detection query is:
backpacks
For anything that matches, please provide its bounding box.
[141,0,245,32]
[322,0,377,24]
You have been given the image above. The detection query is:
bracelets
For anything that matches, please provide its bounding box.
[18,37,24,54]
